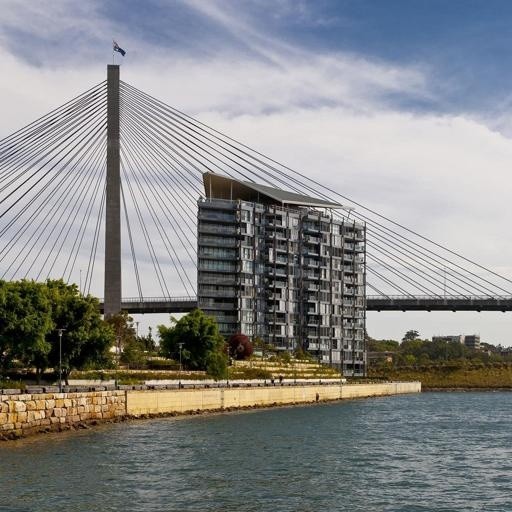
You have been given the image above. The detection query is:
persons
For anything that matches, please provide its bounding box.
[271,375,283,386]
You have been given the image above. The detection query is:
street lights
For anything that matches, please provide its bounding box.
[114,336,121,391]
[54,328,67,393]
[225,343,232,388]
[176,342,186,390]
